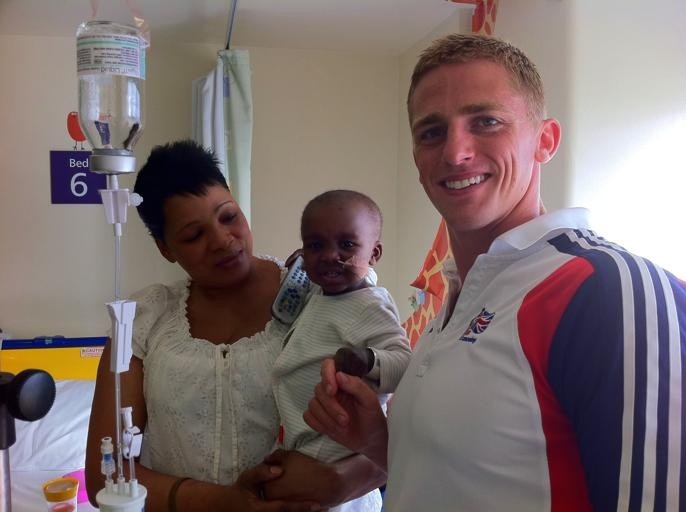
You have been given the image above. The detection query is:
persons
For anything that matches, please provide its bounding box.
[265,187,414,469]
[303,31,686,512]
[81,138,397,511]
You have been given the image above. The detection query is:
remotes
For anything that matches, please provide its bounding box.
[270,255,310,325]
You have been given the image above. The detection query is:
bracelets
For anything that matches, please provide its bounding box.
[167,476,193,512]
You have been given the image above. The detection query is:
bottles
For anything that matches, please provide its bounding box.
[77,18,148,173]
